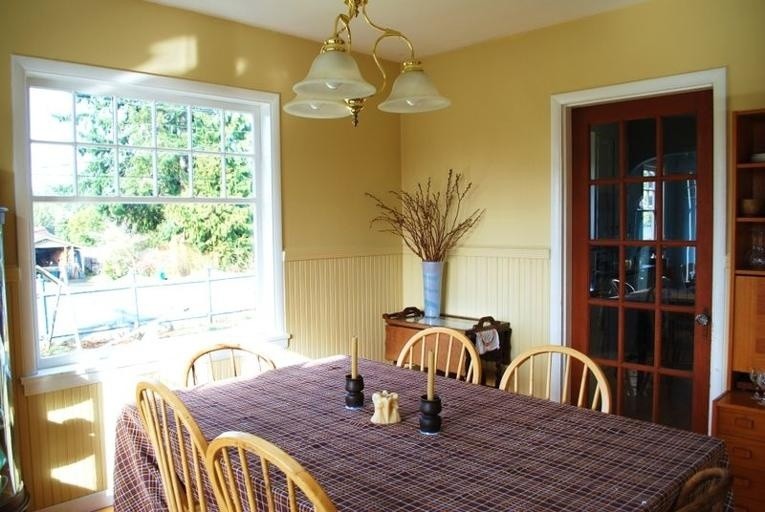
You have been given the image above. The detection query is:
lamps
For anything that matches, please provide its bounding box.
[280,1,452,129]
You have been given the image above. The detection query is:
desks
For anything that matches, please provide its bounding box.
[383,307,513,388]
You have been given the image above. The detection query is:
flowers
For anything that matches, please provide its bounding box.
[364,167,488,260]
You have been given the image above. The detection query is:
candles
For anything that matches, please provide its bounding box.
[427,348,434,400]
[352,335,358,379]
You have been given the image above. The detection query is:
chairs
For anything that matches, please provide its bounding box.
[397,327,612,414]
[125,379,342,512]
[182,342,278,389]
[675,466,732,512]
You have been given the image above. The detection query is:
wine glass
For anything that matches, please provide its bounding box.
[749,368,765,406]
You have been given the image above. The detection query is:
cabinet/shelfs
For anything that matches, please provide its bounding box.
[712,109,765,511]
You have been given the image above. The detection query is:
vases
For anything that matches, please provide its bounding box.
[422,262,444,318]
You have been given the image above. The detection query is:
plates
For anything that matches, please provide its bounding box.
[750,154,765,162]
